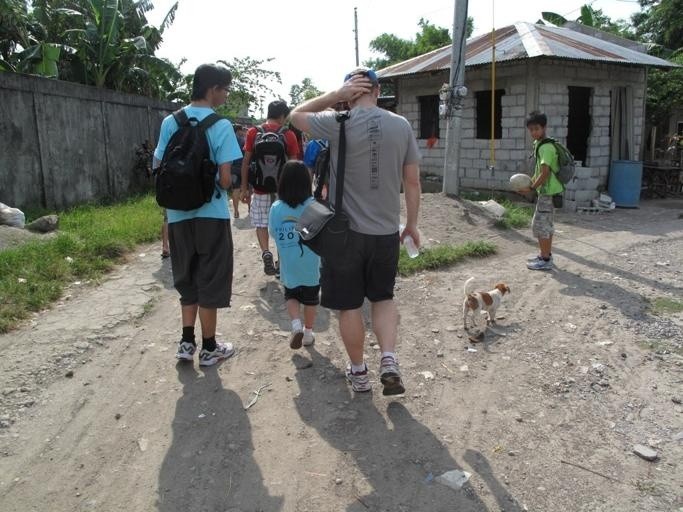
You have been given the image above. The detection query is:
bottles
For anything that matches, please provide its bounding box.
[398,222,419,259]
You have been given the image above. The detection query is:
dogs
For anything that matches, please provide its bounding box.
[461,277,511,330]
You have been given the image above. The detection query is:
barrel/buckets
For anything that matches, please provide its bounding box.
[608,159,645,208]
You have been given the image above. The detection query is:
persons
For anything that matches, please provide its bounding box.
[286,66,423,396]
[515,108,566,272]
[266,158,322,350]
[143,99,343,282]
[146,63,246,368]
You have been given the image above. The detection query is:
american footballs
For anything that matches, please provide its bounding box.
[510,174,533,192]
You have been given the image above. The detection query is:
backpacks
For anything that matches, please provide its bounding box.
[314,138,331,203]
[154,108,227,212]
[246,124,291,193]
[535,136,576,184]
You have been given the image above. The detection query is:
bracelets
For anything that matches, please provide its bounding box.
[528,186,533,191]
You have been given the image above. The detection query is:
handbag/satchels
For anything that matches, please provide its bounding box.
[296,197,351,259]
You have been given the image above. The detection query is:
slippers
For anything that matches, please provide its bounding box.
[161,250,169,257]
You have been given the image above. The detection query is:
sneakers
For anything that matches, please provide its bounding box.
[345,362,371,393]
[262,250,281,279]
[234,211,239,218]
[289,329,304,350]
[174,341,196,361]
[302,333,315,346]
[378,355,406,397]
[525,255,554,271]
[198,342,236,367]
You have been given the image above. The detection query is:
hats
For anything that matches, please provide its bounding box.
[344,69,379,90]
